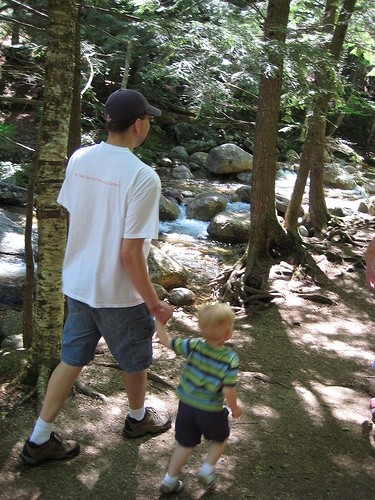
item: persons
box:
[366,235,375,418]
[155,304,243,494]
[19,89,174,466]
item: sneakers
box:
[196,472,217,491]
[19,431,80,468]
[122,407,171,438]
[370,397,375,416]
[160,480,184,494]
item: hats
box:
[104,89,162,123]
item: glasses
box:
[140,115,155,122]
